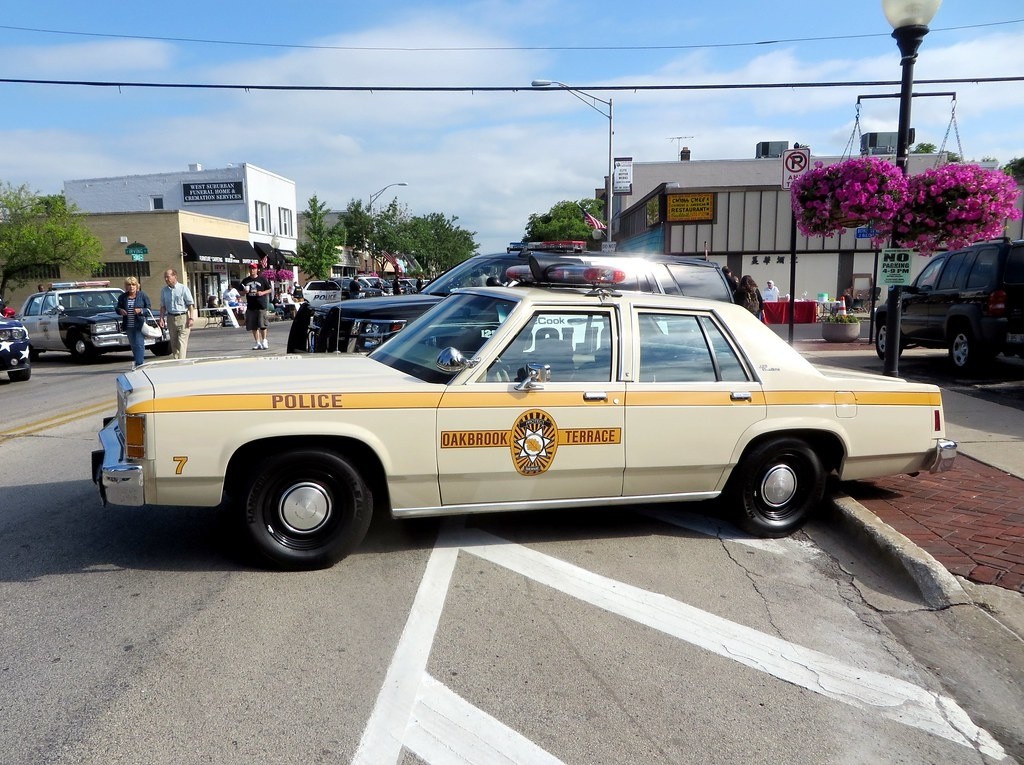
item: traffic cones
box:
[838,296,848,317]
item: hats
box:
[248,262,257,269]
[767,281,774,285]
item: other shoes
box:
[262,341,268,349]
[252,343,262,350]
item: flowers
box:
[260,269,277,281]
[276,268,295,282]
[790,155,912,250]
[894,161,1024,259]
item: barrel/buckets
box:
[818,293,828,301]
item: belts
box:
[168,312,187,316]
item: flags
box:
[578,206,607,231]
[382,251,397,266]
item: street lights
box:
[880,0,941,380]
[370,182,408,274]
[531,80,614,242]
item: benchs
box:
[640,342,731,381]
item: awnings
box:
[182,233,261,264]
[253,242,285,264]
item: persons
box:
[239,264,272,349]
[208,285,241,327]
[348,276,362,298]
[273,281,305,321]
[115,277,151,365]
[38,283,44,292]
[159,269,194,359]
[374,277,424,294]
[722,266,779,322]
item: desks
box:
[816,300,841,323]
[275,301,307,321]
[761,301,817,325]
[199,306,242,330]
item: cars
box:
[355,275,432,296]
[90,281,955,573]
[15,280,173,365]
[0,308,32,382]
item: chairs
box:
[569,346,611,381]
[530,338,574,381]
[206,303,223,329]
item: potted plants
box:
[822,313,864,342]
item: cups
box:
[245,286,250,293]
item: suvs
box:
[290,245,737,376]
[876,237,1024,380]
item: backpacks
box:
[294,285,302,295]
[742,290,759,313]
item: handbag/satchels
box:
[141,308,162,337]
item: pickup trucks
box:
[279,279,383,314]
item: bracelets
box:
[189,317,194,320]
